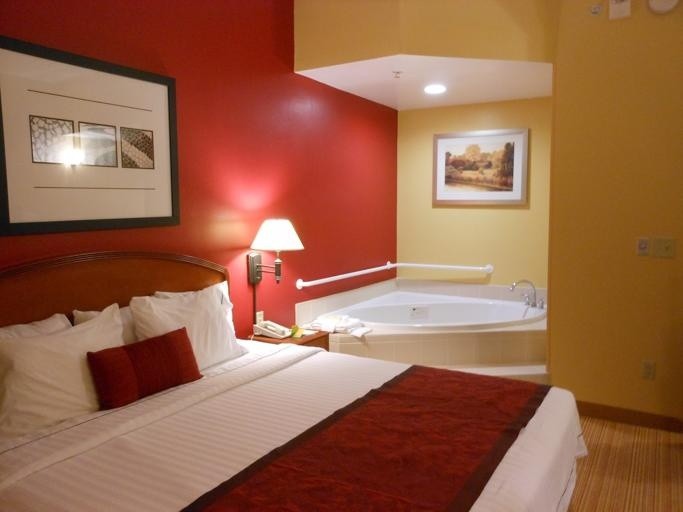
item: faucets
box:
[510,279,538,308]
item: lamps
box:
[249,219,305,281]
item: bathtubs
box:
[303,290,549,370]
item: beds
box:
[0,250,588,512]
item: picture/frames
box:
[0,36,180,237]
[432,127,529,205]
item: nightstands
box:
[247,329,329,352]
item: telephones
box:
[251,318,295,339]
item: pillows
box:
[154,280,235,340]
[130,287,249,371]
[0,313,72,342]
[72,306,138,345]
[0,303,125,438]
[87,327,204,410]
